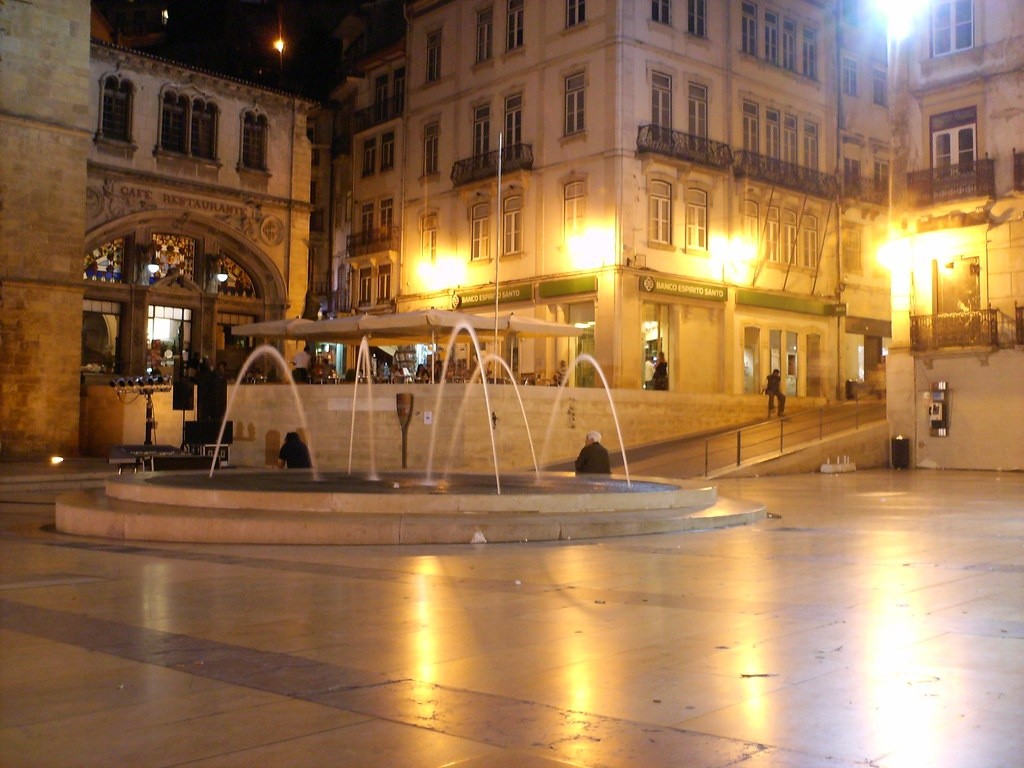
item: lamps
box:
[217,260,228,282]
[147,249,159,273]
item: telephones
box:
[929,403,943,421]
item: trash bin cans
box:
[846,378,858,399]
[891,434,910,471]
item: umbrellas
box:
[231,306,583,385]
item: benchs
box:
[108,444,177,475]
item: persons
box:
[645,351,667,390]
[288,345,314,382]
[390,365,401,383]
[416,364,429,384]
[760,369,786,417]
[575,431,610,473]
[277,432,313,469]
[434,360,447,382]
[556,360,569,387]
